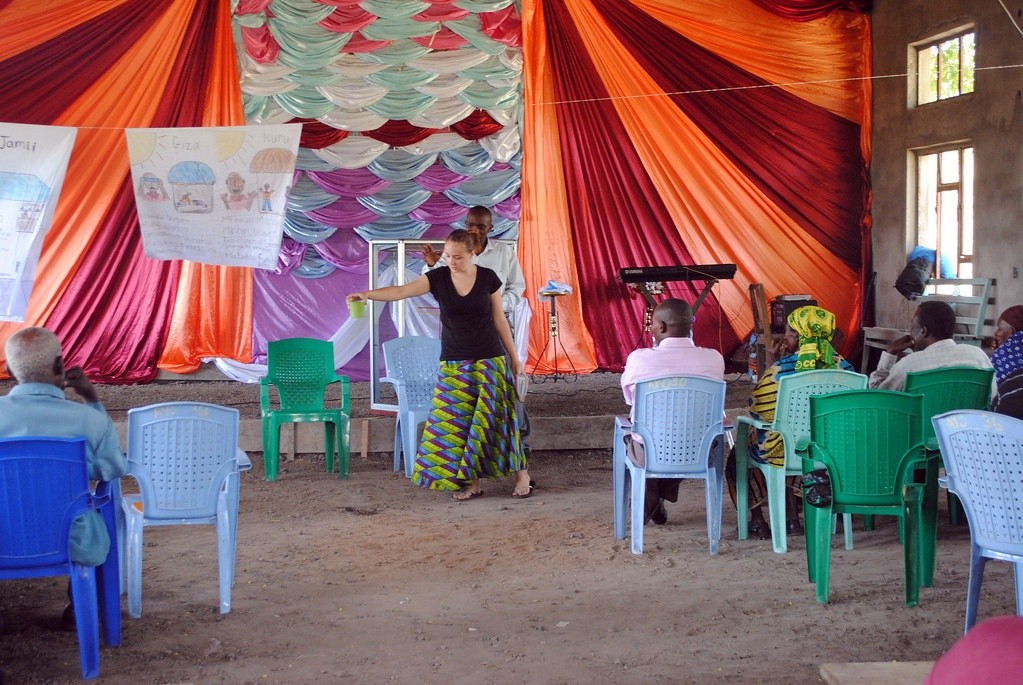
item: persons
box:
[0,327,130,632]
[992,306,1023,382]
[345,229,537,501]
[726,306,856,539]
[868,301,1000,483]
[620,299,725,525]
[421,205,532,456]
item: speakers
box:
[769,299,818,333]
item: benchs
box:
[860,278,993,377]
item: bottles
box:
[749,352,757,378]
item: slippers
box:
[514,480,536,499]
[451,487,484,502]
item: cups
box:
[349,302,364,318]
[753,374,758,383]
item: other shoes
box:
[61,606,78,632]
[644,496,660,525]
[786,519,803,537]
[650,500,667,523]
[736,520,769,538]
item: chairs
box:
[736,366,1023,638]
[117,400,253,615]
[258,338,352,480]
[612,372,737,555]
[382,335,443,477]
[0,438,121,678]
